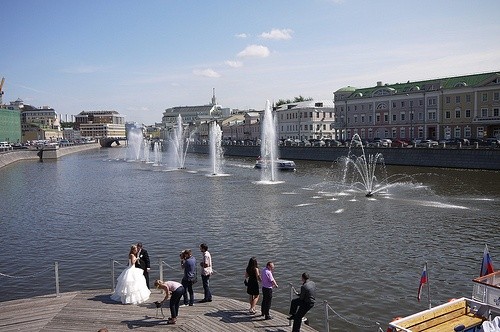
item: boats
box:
[255,155,296,172]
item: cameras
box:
[180,251,185,259]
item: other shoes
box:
[250,309,256,314]
[169,317,177,320]
[265,316,272,320]
[168,320,176,324]
[200,298,212,303]
[190,303,193,306]
[287,316,296,320]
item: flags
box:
[417,260,428,303]
[479,244,494,278]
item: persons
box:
[179,249,197,306]
[154,280,184,324]
[286,271,315,332]
[261,262,278,320]
[244,256,263,313]
[200,243,213,302]
[134,243,151,289]
[110,243,151,305]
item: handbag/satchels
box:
[203,267,213,276]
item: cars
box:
[185,137,500,149]
[0,137,94,149]
